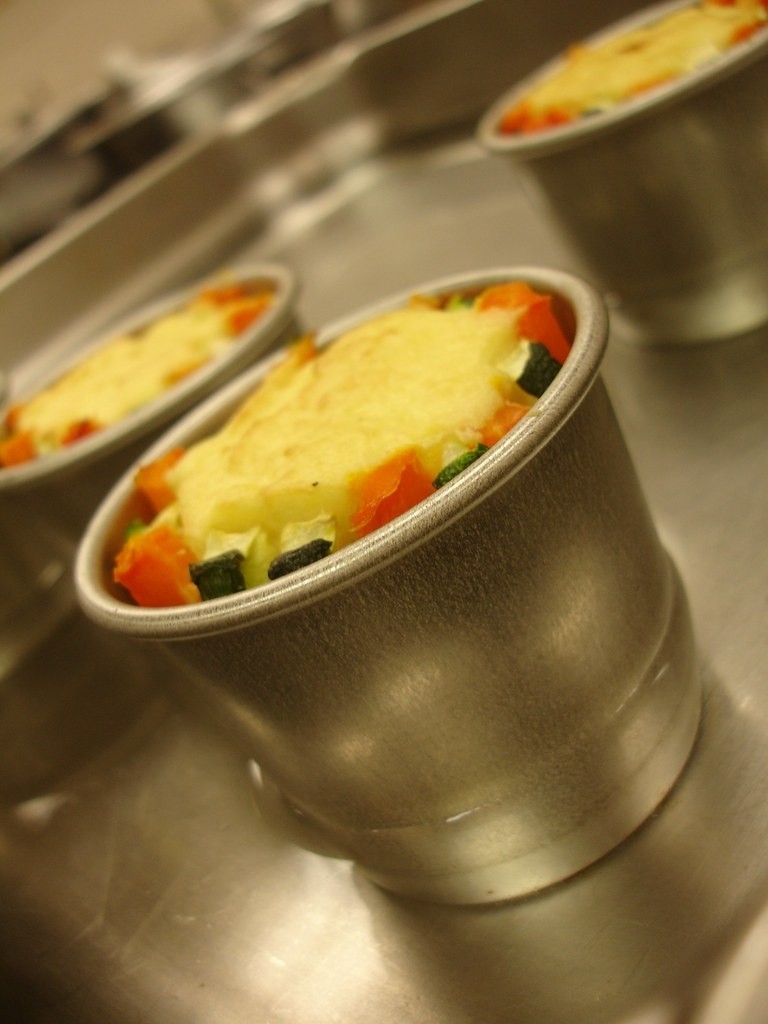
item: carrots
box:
[492,0,768,139]
[1,278,570,606]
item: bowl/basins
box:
[473,0,767,343]
[72,265,676,837]
[2,265,302,538]
